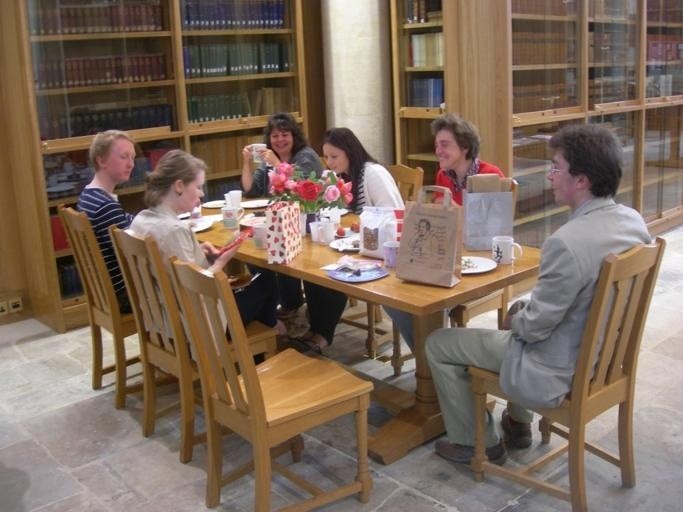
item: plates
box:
[461,253,498,276]
[330,238,361,252]
[330,265,387,283]
[177,200,224,233]
[333,227,360,237]
[241,199,347,227]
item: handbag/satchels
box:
[264,196,304,265]
[394,182,466,290]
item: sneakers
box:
[500,405,533,449]
[433,436,508,466]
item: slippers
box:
[286,330,332,359]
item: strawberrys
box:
[351,223,359,232]
[336,228,345,236]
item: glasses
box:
[548,167,571,175]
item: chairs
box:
[338,165,425,359]
[107,223,280,464]
[56,203,179,409]
[391,177,519,376]
[166,254,375,512]
[469,235,667,512]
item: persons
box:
[81,128,136,314]
[129,149,311,367]
[242,115,324,320]
[287,127,406,357]
[408,219,439,263]
[382,113,506,355]
[425,121,652,465]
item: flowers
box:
[267,163,353,214]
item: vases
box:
[306,214,316,233]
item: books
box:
[26,0,291,138]
[46,149,168,297]
[409,1,681,216]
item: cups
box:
[253,223,270,249]
[252,142,268,161]
[317,223,335,244]
[491,235,523,265]
[219,206,244,229]
[383,240,400,267]
[223,190,242,207]
[309,222,318,241]
